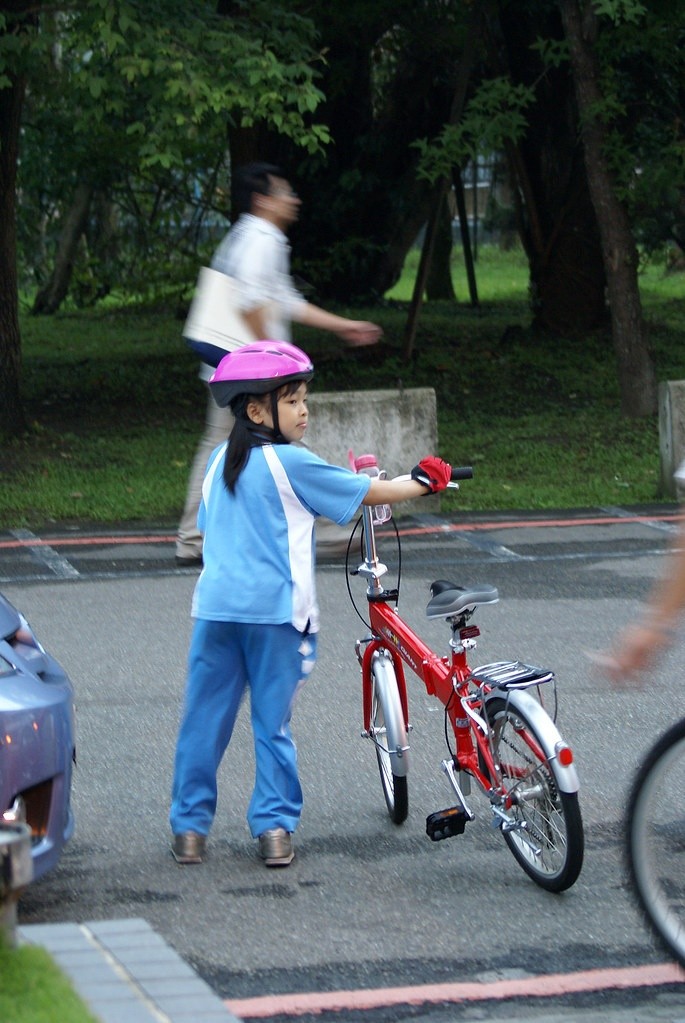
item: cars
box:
[0,590,77,894]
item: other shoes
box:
[174,554,207,568]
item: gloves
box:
[411,455,452,496]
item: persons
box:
[175,161,384,564]
[168,341,454,865]
[608,457,685,674]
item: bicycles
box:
[623,712,685,970]
[344,449,587,895]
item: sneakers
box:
[169,830,207,863]
[254,827,295,865]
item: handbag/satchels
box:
[182,266,260,368]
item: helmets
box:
[208,339,316,408]
